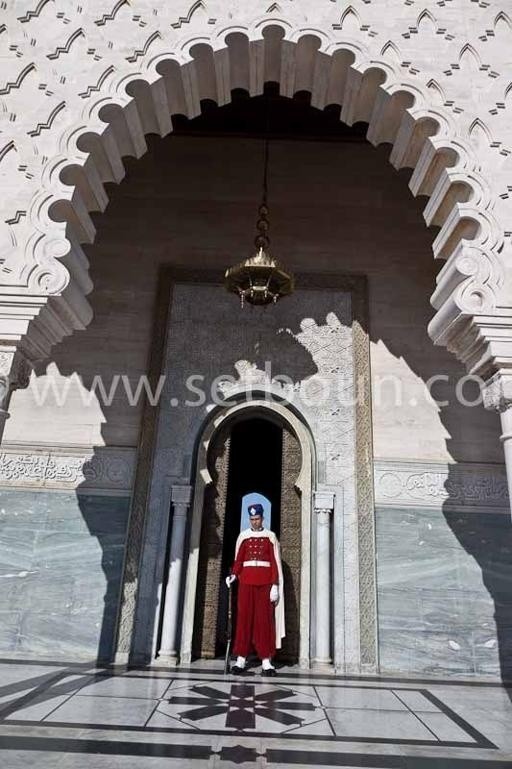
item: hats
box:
[248,504,263,516]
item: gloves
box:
[225,574,236,588]
[269,583,279,603]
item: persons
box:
[225,503,286,677]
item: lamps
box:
[224,139,293,308]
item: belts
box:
[242,560,271,568]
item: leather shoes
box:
[231,665,248,675]
[262,667,276,677]
